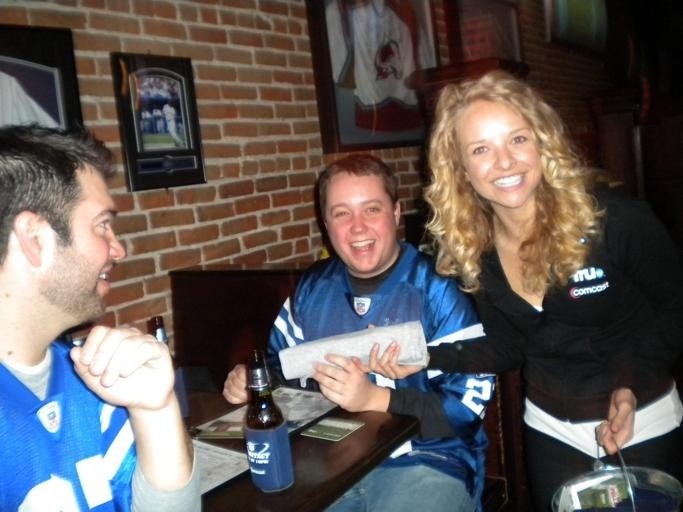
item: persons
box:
[224,153,497,512]
[141,102,177,132]
[374,71,683,512]
[1,122,202,512]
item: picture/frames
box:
[110,53,206,193]
[1,23,84,136]
[305,0,443,155]
[543,0,610,62]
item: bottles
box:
[152,316,190,421]
[241,349,295,495]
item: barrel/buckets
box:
[551,424,682,511]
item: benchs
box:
[168,267,526,512]
